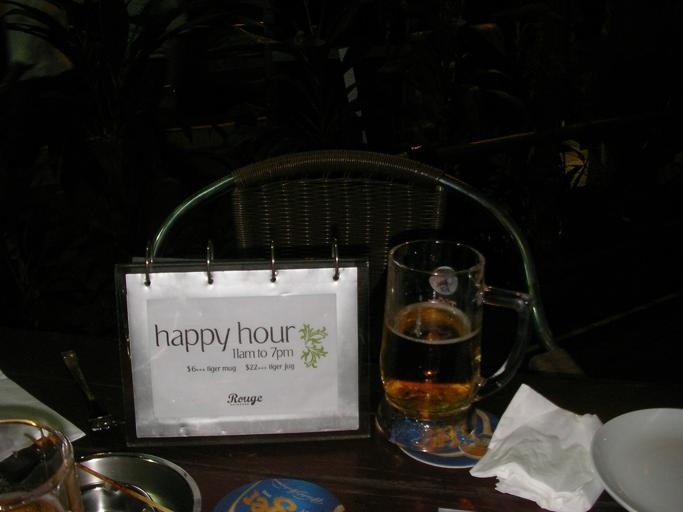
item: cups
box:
[380,238,533,424]
[0,417,84,512]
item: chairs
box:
[151,150,556,376]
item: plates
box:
[591,405,683,512]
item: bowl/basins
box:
[75,450,202,512]
